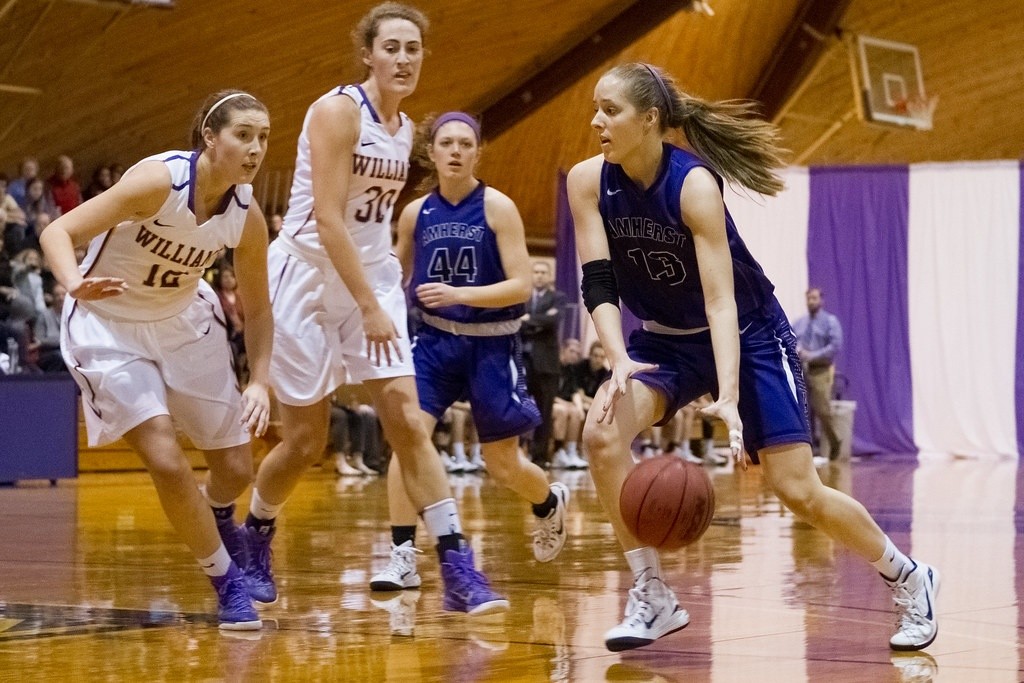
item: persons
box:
[0,154,841,477]
[236,6,513,620]
[367,114,570,594]
[43,89,283,632]
[561,63,941,652]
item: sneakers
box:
[533,481,570,562]
[219,519,250,572]
[440,539,510,615]
[240,523,278,606]
[205,561,261,630]
[604,566,690,652]
[370,540,425,589]
[334,439,726,475]
[879,555,940,650]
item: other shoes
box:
[828,437,844,461]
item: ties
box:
[536,290,540,311]
[802,319,813,349]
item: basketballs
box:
[618,454,718,550]
